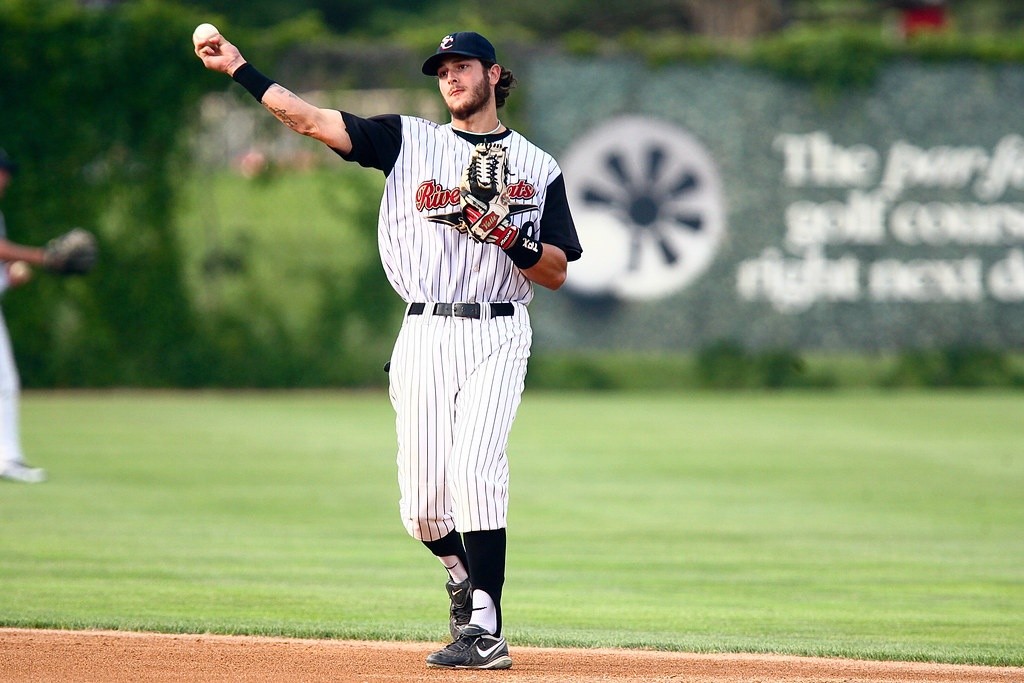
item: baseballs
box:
[193,22,218,47]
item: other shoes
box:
[1,461,46,486]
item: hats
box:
[0,146,26,172]
[421,31,500,76]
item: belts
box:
[407,302,515,320]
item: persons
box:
[194,24,585,673]
[0,146,100,483]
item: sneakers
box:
[422,624,512,671]
[444,575,475,640]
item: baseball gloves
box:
[43,227,97,275]
[461,141,517,245]
